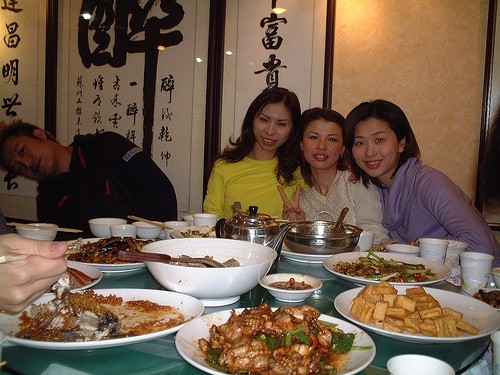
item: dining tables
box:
[0,256,493,375]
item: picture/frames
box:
[0,0,336,225]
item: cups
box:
[183,213,218,226]
[357,230,375,251]
[418,237,499,297]
[386,330,500,375]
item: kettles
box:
[215,202,296,271]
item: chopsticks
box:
[7,221,83,232]
[128,215,173,230]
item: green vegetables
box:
[336,251,437,282]
[203,319,373,375]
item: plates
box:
[279,241,360,263]
[45,262,104,292]
[2,288,205,351]
[171,226,215,238]
[334,286,499,345]
[323,252,452,288]
[175,308,376,375]
[65,238,157,273]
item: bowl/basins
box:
[384,243,420,256]
[89,218,188,238]
[280,219,364,254]
[259,273,323,302]
[141,237,278,307]
[16,222,58,240]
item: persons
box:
[342,99,500,268]
[0,118,178,231]
[203,86,310,220]
[0,233,70,313]
[277,107,390,246]
